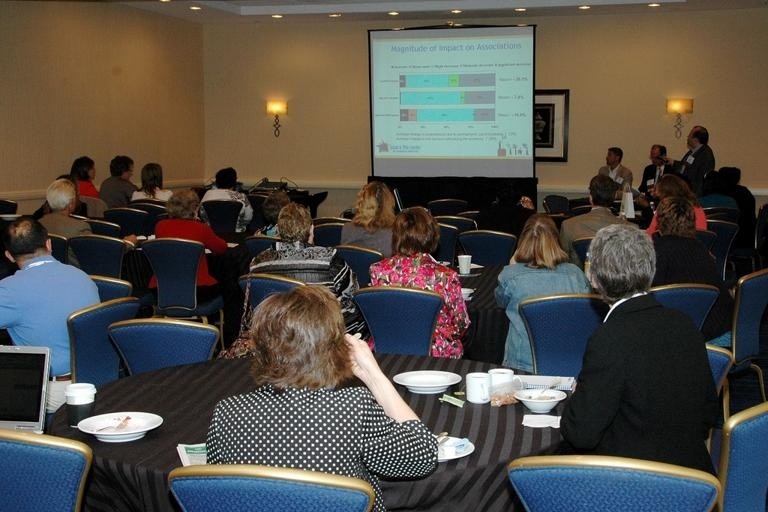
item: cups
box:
[457,254,474,276]
[466,369,524,403]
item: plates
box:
[392,370,462,394]
[431,432,478,464]
[77,411,165,443]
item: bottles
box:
[581,250,593,283]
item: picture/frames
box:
[533,89,570,164]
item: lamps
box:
[264,98,289,139]
[665,95,693,141]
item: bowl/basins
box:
[65,383,96,405]
[515,389,567,414]
[459,286,475,301]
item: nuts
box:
[491,394,518,407]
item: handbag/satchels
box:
[619,183,635,219]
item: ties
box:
[654,168,663,191]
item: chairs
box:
[0,169,766,511]
